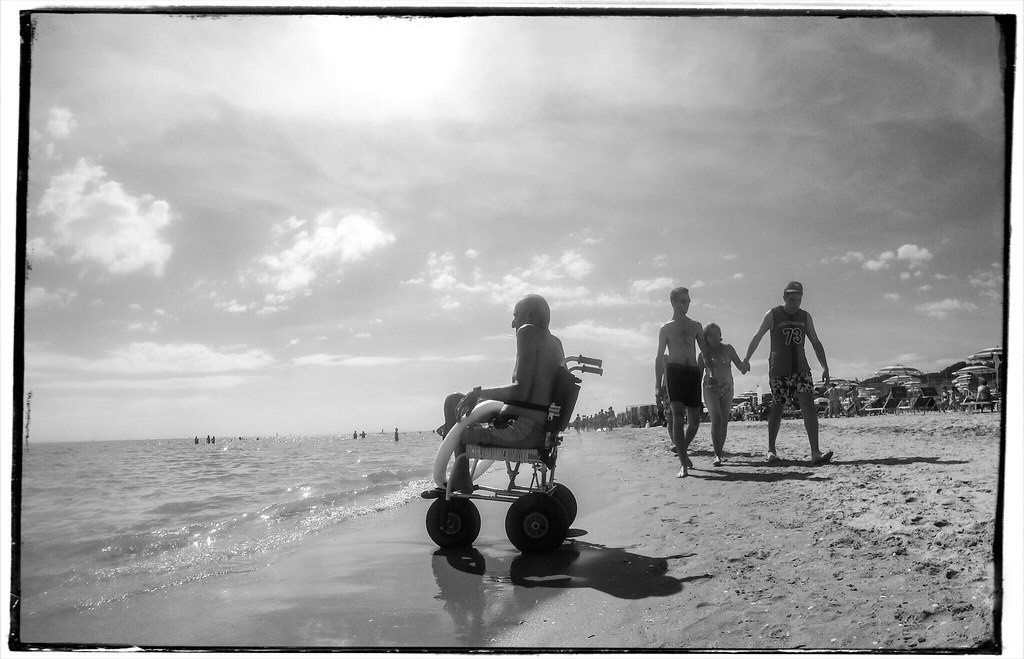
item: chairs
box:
[865,386,908,416]
[960,388,993,412]
[899,386,939,416]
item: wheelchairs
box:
[426,354,603,556]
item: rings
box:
[457,408,462,412]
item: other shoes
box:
[670,443,678,457]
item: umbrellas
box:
[873,363,926,397]
[951,345,1002,399]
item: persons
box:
[420,295,569,500]
[655,286,718,478]
[655,354,692,455]
[568,404,667,434]
[812,381,909,418]
[352,427,436,442]
[700,383,803,422]
[697,321,751,465]
[193,433,260,445]
[916,380,1000,412]
[740,281,834,467]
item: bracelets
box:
[473,385,483,401]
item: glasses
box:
[680,299,690,303]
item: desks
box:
[991,393,1002,411]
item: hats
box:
[784,281,803,293]
